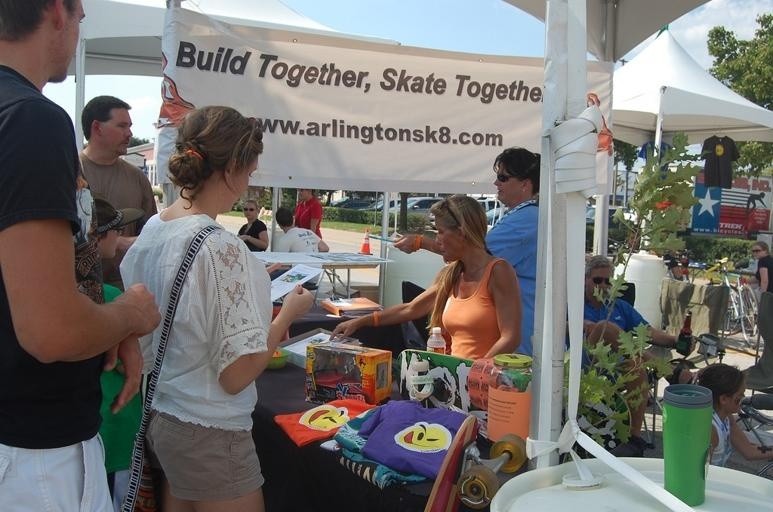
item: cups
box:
[661,386,713,506]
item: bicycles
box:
[706,258,761,350]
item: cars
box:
[584,203,627,224]
[330,197,510,222]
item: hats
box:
[89,197,147,236]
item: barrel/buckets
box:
[614,253,664,329]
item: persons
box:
[119,106,315,511]
[295,188,322,238]
[77,95,157,290]
[329,198,523,359]
[698,364,772,468]
[752,241,773,295]
[394,148,540,354]
[1,1,162,511]
[266,209,329,288]
[566,256,695,449]
[237,201,268,252]
[94,197,142,509]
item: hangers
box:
[703,126,734,146]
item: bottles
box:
[427,327,446,354]
[360,228,371,255]
[676,310,693,355]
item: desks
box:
[255,253,392,308]
[288,298,384,342]
[246,360,526,512]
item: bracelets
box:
[415,237,422,250]
[372,311,380,327]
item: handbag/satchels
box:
[121,436,159,512]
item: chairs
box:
[401,281,431,344]
[739,288,772,451]
[616,283,659,448]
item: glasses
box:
[751,248,764,253]
[242,207,254,212]
[496,173,515,183]
[590,275,613,285]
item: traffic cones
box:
[358,228,374,255]
